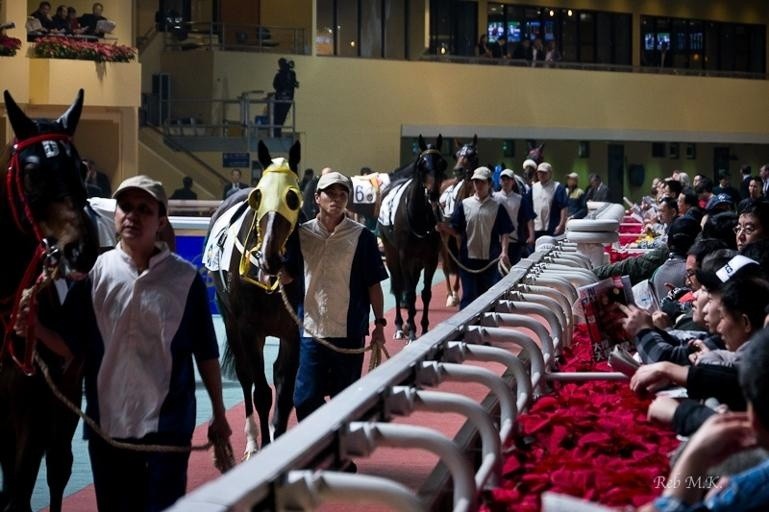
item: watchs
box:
[373,317,387,328]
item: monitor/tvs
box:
[524,19,556,43]
[675,32,704,52]
[644,32,672,51]
[488,18,521,44]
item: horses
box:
[302,159,418,223]
[0,87,177,512]
[203,138,305,461]
[440,133,480,308]
[376,134,448,344]
[521,142,545,187]
[487,161,530,195]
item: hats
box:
[500,158,581,181]
[316,171,351,193]
[471,167,491,181]
[112,174,167,204]
[666,193,760,284]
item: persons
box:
[270,55,300,133]
[585,173,613,201]
[523,159,541,188]
[84,161,110,197]
[297,166,387,261]
[491,168,538,267]
[434,166,516,311]
[165,176,197,200]
[278,171,389,424]
[585,159,768,512]
[566,172,590,220]
[11,170,235,510]
[21,2,113,42]
[223,169,248,201]
[525,161,571,255]
[401,23,723,71]
